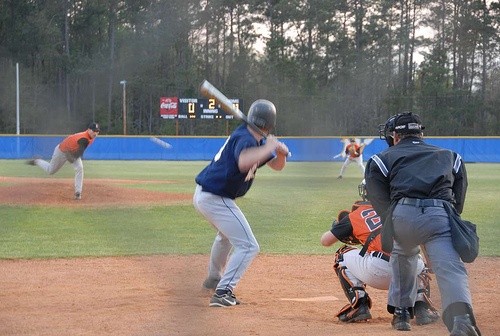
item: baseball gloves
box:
[349,146,355,153]
[330,208,363,245]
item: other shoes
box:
[338,176,342,179]
[390,307,411,332]
[25,158,37,165]
[449,314,481,336]
[73,192,82,200]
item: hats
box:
[388,111,425,137]
[349,138,355,142]
[88,122,100,131]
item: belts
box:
[398,197,451,208]
[373,251,390,262]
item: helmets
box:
[247,98,276,129]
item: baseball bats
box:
[198,79,292,158]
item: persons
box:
[320,180,440,324]
[364,112,482,336]
[337,137,365,179]
[193,99,289,306]
[26,122,100,200]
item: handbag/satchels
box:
[380,201,397,252]
[442,202,480,263]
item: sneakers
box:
[414,301,441,326]
[202,278,222,290]
[209,290,240,307]
[338,297,372,323]
